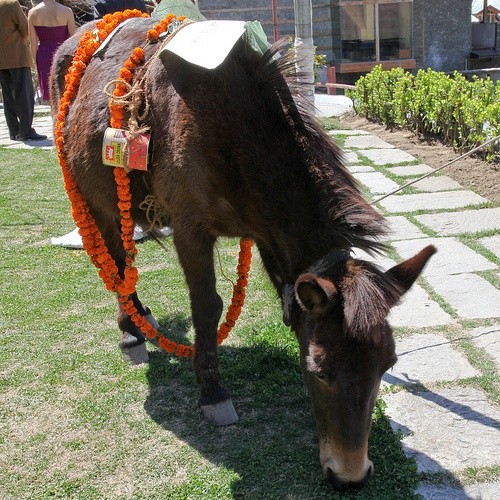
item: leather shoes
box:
[16,131,48,141]
[9,132,19,139]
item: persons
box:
[0,1,48,141]
[27,0,77,101]
[150,0,207,22]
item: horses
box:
[47,15,439,493]
